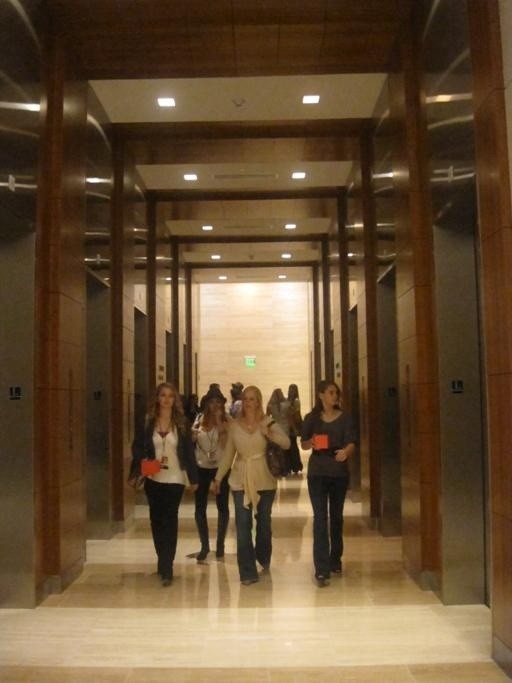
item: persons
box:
[300,380,357,588]
[210,385,292,585]
[184,384,304,491]
[190,388,234,560]
[131,382,199,588]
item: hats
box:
[232,383,243,390]
[201,388,226,409]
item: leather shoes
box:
[242,562,270,584]
[158,568,171,586]
[316,567,341,579]
[196,550,209,560]
[216,543,224,556]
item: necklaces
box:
[157,416,172,449]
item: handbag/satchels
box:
[266,420,292,477]
